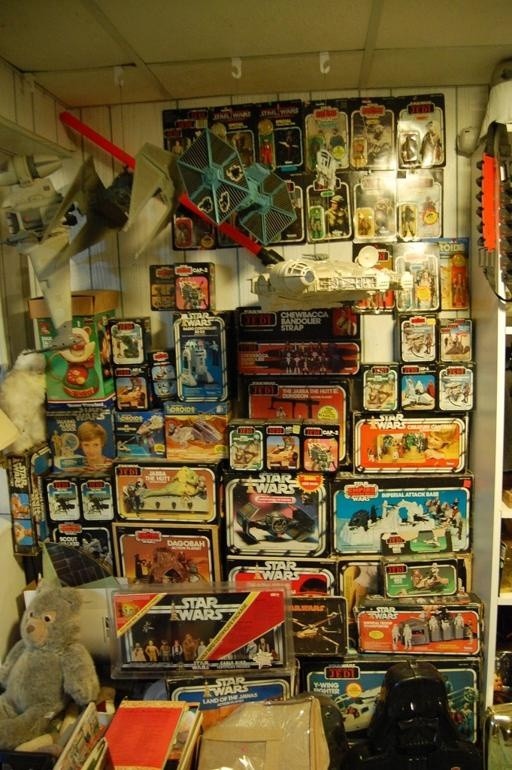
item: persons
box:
[424,423,459,464]
[132,635,271,664]
[82,425,116,472]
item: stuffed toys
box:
[1,581,101,752]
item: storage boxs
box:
[53,700,201,769]
